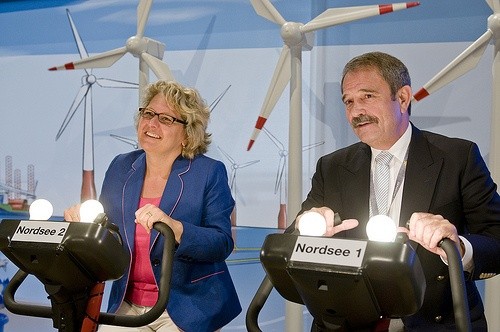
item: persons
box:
[64,82,242,332]
[284,51,500,332]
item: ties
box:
[370,153,405,214]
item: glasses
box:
[138,107,185,126]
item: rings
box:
[146,211,151,216]
[70,213,78,217]
